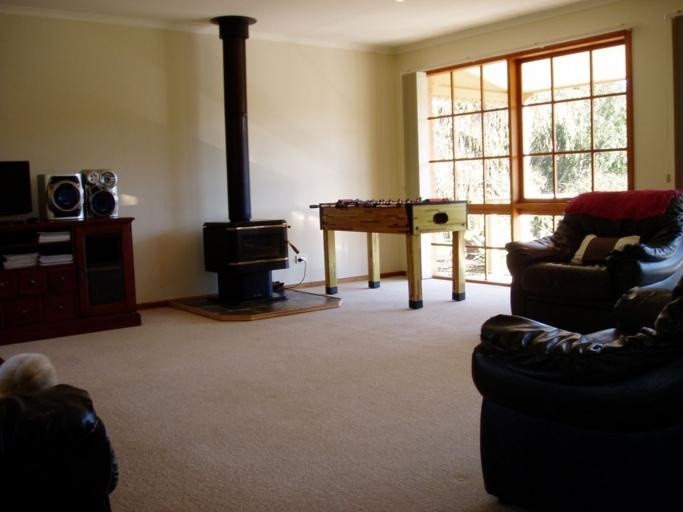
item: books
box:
[3,253,75,270]
[37,232,70,243]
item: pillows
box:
[571,234,641,267]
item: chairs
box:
[471,268,683,509]
[503,188,683,339]
[0,353,121,512]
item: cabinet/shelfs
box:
[1,218,143,345]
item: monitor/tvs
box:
[0,160,34,217]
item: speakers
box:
[37,172,84,221]
[79,168,120,219]
